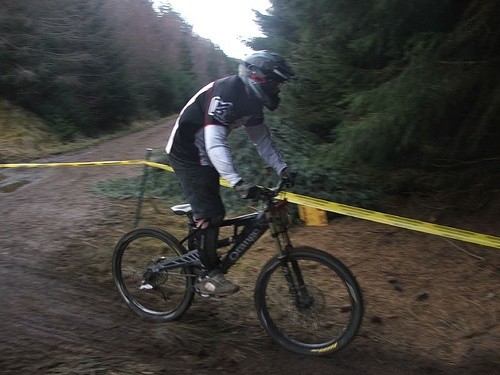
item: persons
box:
[166,49,301,297]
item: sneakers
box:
[199,273,240,296]
[180,265,206,277]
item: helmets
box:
[237,50,294,112]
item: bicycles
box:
[112,169,364,357]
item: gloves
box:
[234,180,261,201]
[281,167,295,188]
